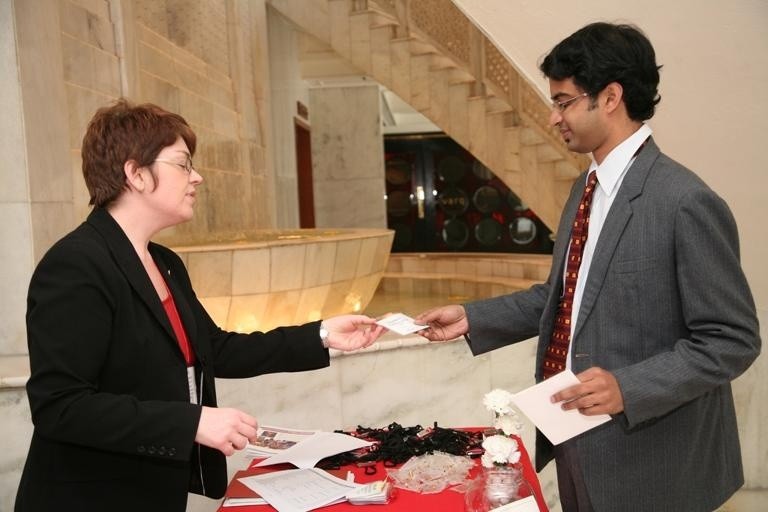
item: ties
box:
[541,171,598,382]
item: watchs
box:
[319,320,330,348]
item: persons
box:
[14,96,393,512]
[413,22,761,512]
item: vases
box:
[482,471,520,507]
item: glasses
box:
[550,92,590,112]
[154,156,195,173]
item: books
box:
[246,426,322,457]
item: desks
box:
[215,426,547,511]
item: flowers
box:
[480,434,522,470]
[482,387,525,436]
